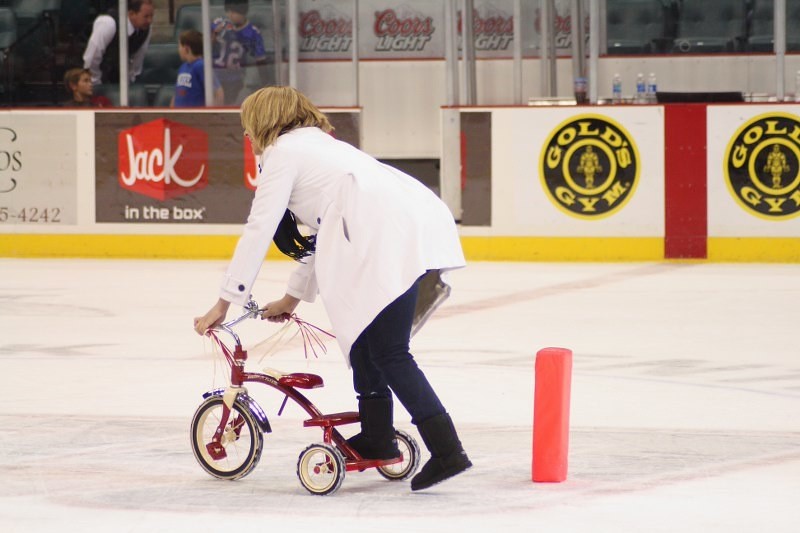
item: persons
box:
[63,0,155,107]
[210,0,274,105]
[193,86,476,491]
[169,29,225,108]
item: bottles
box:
[648,73,658,104]
[636,74,646,104]
[613,73,622,104]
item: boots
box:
[334,396,400,460]
[411,413,473,491]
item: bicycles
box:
[187,293,421,497]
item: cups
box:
[574,77,586,104]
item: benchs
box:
[0,1,287,111]
[605,0,800,54]
